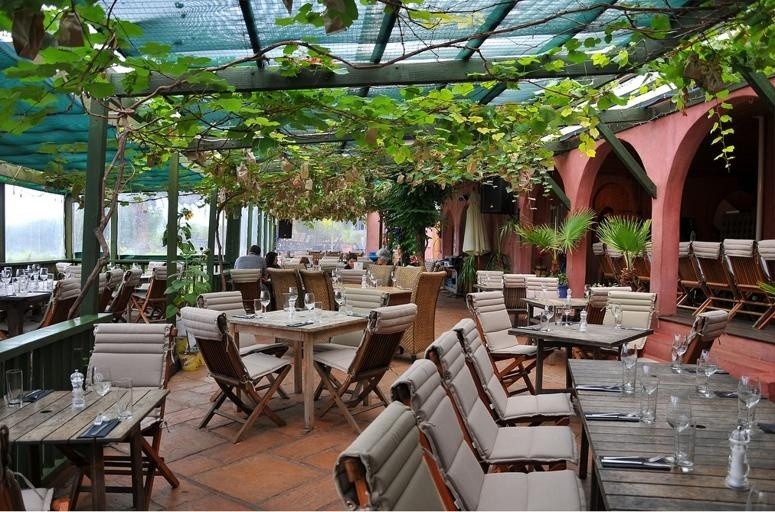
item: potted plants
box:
[512,207,597,287]
[591,213,657,293]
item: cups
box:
[40,268,48,290]
[738,399,756,436]
[32,263,40,278]
[12,277,20,294]
[620,341,638,395]
[391,271,397,288]
[47,273,53,290]
[283,293,290,311]
[672,343,682,370]
[114,375,134,421]
[563,301,571,328]
[332,269,338,289]
[544,302,554,333]
[696,358,707,393]
[666,394,688,466]
[637,360,660,424]
[640,383,657,421]
[27,265,34,286]
[541,312,549,332]
[93,367,111,424]
[304,292,316,323]
[668,329,690,373]
[699,349,719,399]
[623,359,636,394]
[260,290,270,318]
[556,307,563,328]
[254,299,262,315]
[335,290,344,316]
[611,300,620,326]
[314,301,322,321]
[6,369,24,408]
[288,287,298,302]
[678,417,696,473]
[16,268,25,289]
[2,269,11,294]
[737,376,762,439]
[5,266,12,280]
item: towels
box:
[575,382,621,391]
[584,409,641,422]
[232,312,256,319]
[600,453,674,471]
[286,319,314,327]
[757,421,775,434]
[517,324,543,331]
[7,387,55,403]
[684,365,729,376]
[713,389,739,398]
[623,325,651,333]
[347,310,368,318]
[76,416,120,439]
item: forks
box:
[575,385,626,392]
[603,451,669,463]
[578,382,619,389]
[592,410,640,418]
[29,390,44,399]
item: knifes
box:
[91,420,113,436]
[25,389,41,397]
[599,458,679,468]
[584,414,642,420]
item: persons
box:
[344,254,360,270]
[264,252,280,267]
[235,245,267,279]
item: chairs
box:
[197,290,291,403]
[635,309,730,363]
[332,400,459,510]
[572,287,633,358]
[581,290,658,360]
[71,323,181,511]
[313,287,391,406]
[265,251,374,271]
[627,241,651,293]
[466,291,554,396]
[502,274,536,328]
[338,270,365,284]
[390,359,590,510]
[99,273,107,313]
[676,242,722,316]
[299,268,339,311]
[125,266,182,322]
[593,243,620,286]
[524,276,563,350]
[362,265,395,288]
[230,269,270,314]
[607,242,647,292]
[0,330,11,342]
[131,261,167,309]
[723,239,775,329]
[423,330,578,472]
[692,241,756,320]
[181,306,293,444]
[62,265,81,279]
[104,268,143,323]
[452,317,576,427]
[646,242,651,264]
[100,268,123,313]
[0,424,73,511]
[54,263,74,280]
[393,266,422,290]
[267,267,305,311]
[313,303,418,436]
[177,262,186,278]
[391,271,447,364]
[476,270,504,292]
[756,240,775,330]
[37,278,81,330]
